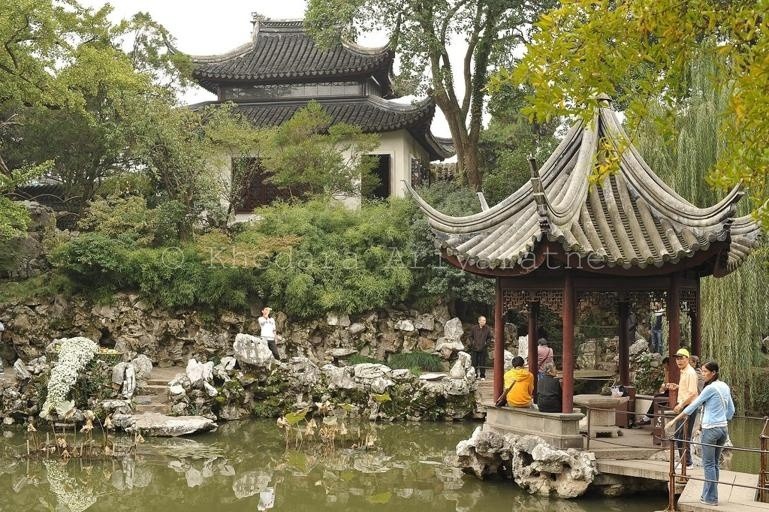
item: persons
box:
[664,361,735,506]
[537,338,553,378]
[651,301,666,356]
[666,348,697,470]
[537,363,561,412]
[468,316,491,382]
[258,305,281,362]
[504,355,534,409]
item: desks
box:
[574,394,629,437]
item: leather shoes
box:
[636,419,651,425]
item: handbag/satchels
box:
[495,394,507,406]
[691,430,733,468]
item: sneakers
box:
[674,462,693,469]
[700,495,718,506]
[481,378,485,381]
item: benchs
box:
[481,402,584,441]
[635,395,655,424]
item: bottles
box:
[657,383,673,393]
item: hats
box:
[674,349,690,358]
